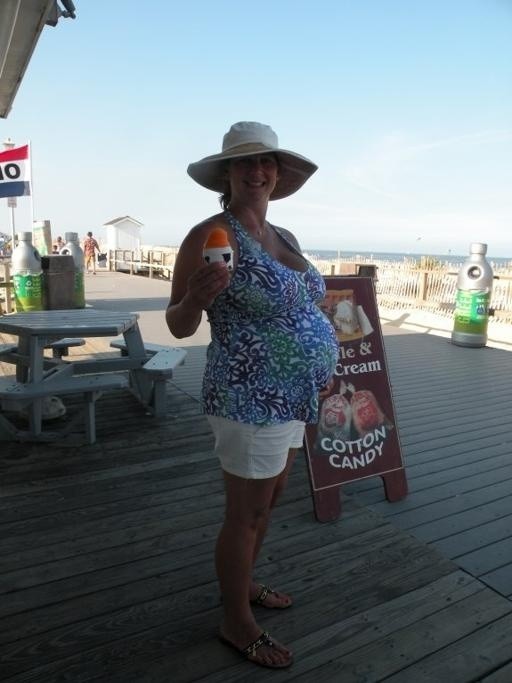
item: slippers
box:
[249,583,292,610]
[216,629,294,668]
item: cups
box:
[202,248,233,273]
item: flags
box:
[0,144,31,198]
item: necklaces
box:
[231,208,268,236]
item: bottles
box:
[59,232,87,309]
[10,233,47,312]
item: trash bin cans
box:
[95,252,107,268]
[12,232,85,313]
[339,263,379,288]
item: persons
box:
[164,121,338,669]
[0,229,100,274]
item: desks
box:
[0,309,152,439]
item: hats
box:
[187,121,318,200]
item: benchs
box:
[0,336,188,444]
